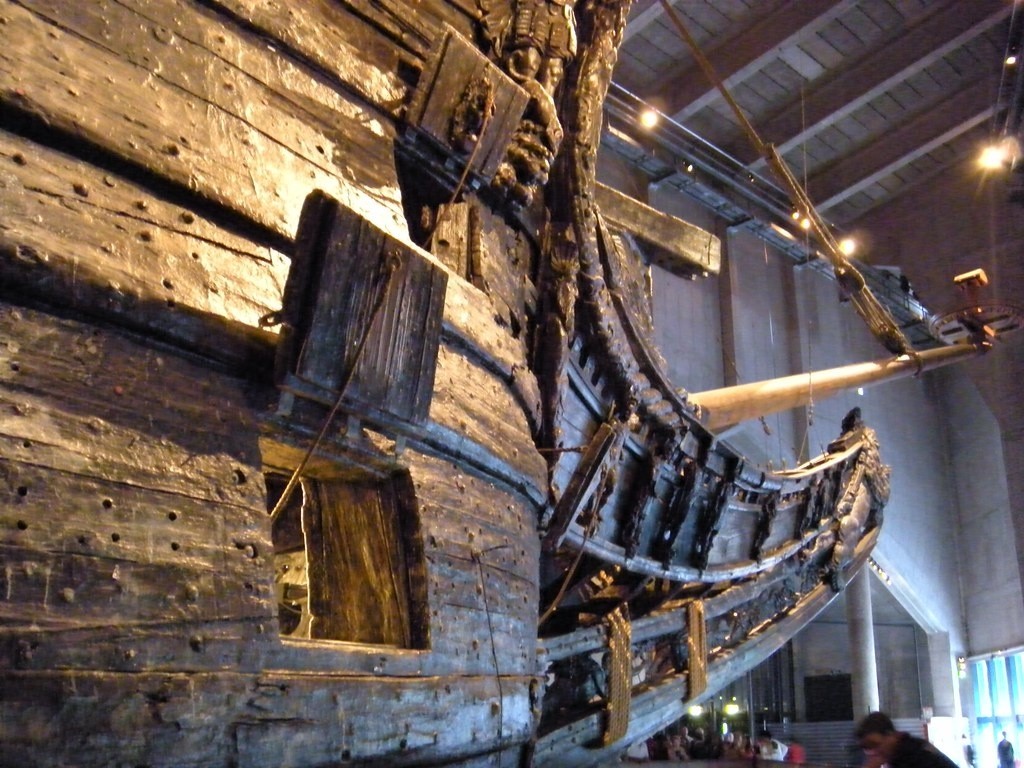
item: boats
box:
[0,1,1008,768]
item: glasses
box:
[858,737,884,750]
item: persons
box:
[626,725,806,768]
[853,711,1015,768]
